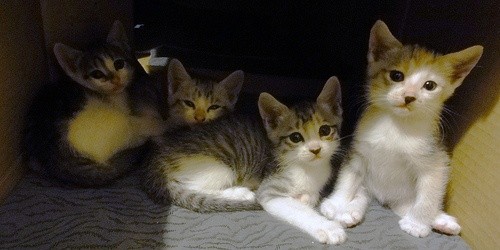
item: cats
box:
[138,75,348,246]
[318,19,485,238]
[27,18,167,189]
[168,58,311,129]
